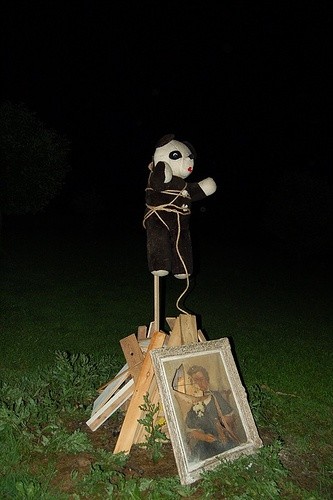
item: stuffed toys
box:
[146,133,217,279]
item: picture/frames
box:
[147,336,263,487]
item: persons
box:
[183,364,235,462]
